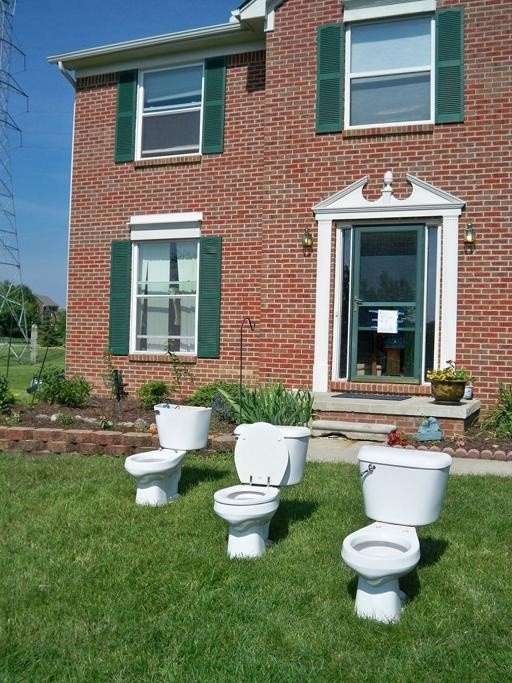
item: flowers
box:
[425,361,468,380]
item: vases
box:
[430,380,466,403]
[465,386,473,399]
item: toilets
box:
[341,443,452,624]
[213,420,311,559]
[124,401,212,509]
